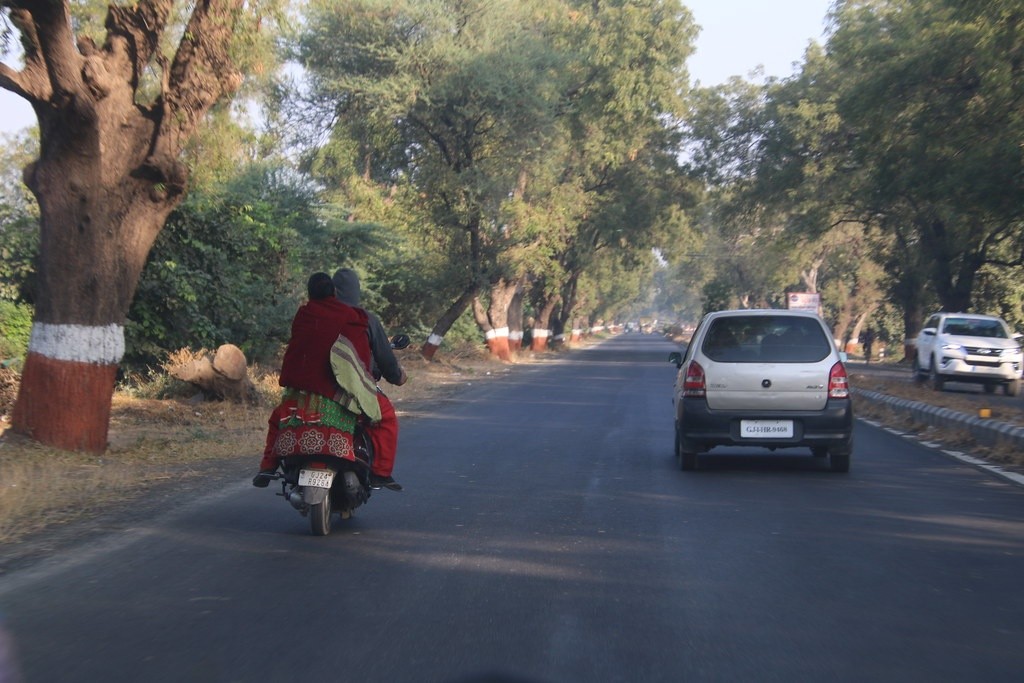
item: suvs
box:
[912,311,1024,398]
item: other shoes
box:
[371,474,402,492]
[253,470,274,488]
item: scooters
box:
[259,333,411,536]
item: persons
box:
[254,268,407,493]
[860,327,873,354]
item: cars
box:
[668,308,854,473]
